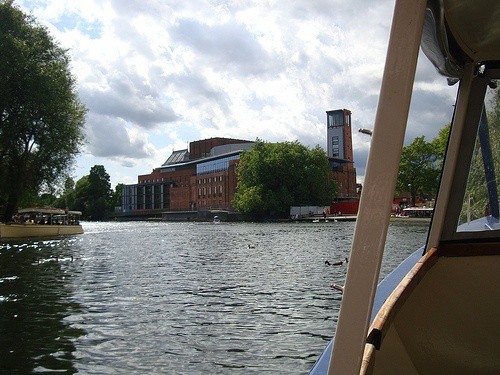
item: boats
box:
[0,208,83,239]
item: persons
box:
[321,208,342,217]
[11,214,79,226]
